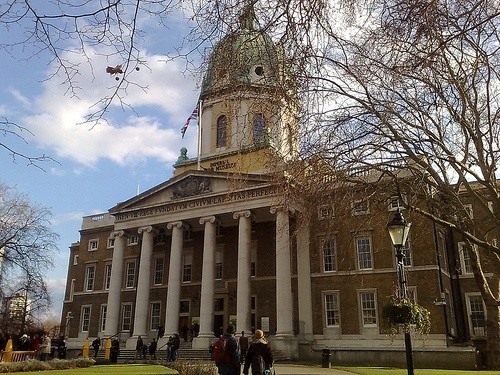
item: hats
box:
[226,326,235,333]
[242,331,244,333]
[254,330,264,338]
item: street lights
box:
[386,207,414,375]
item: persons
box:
[92,337,102,358]
[110,337,120,364]
[167,332,180,362]
[148,338,157,362]
[243,329,275,375]
[190,320,199,342]
[136,336,148,359]
[210,325,246,375]
[239,331,248,364]
[38,334,52,361]
[156,323,165,342]
[15,334,32,350]
[181,324,189,342]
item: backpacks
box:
[252,355,264,374]
[213,338,230,363]
